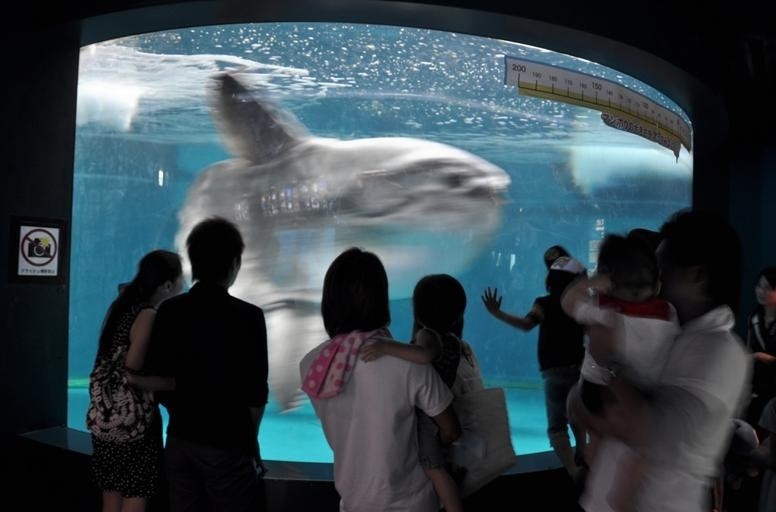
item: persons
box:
[558,206,748,512]
[477,244,593,481]
[138,214,271,511]
[83,248,183,511]
[356,270,475,510]
[294,244,462,512]
[721,266,775,512]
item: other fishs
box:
[75,44,330,134]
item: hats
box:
[544,244,590,302]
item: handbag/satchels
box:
[442,388,518,511]
[86,355,159,447]
